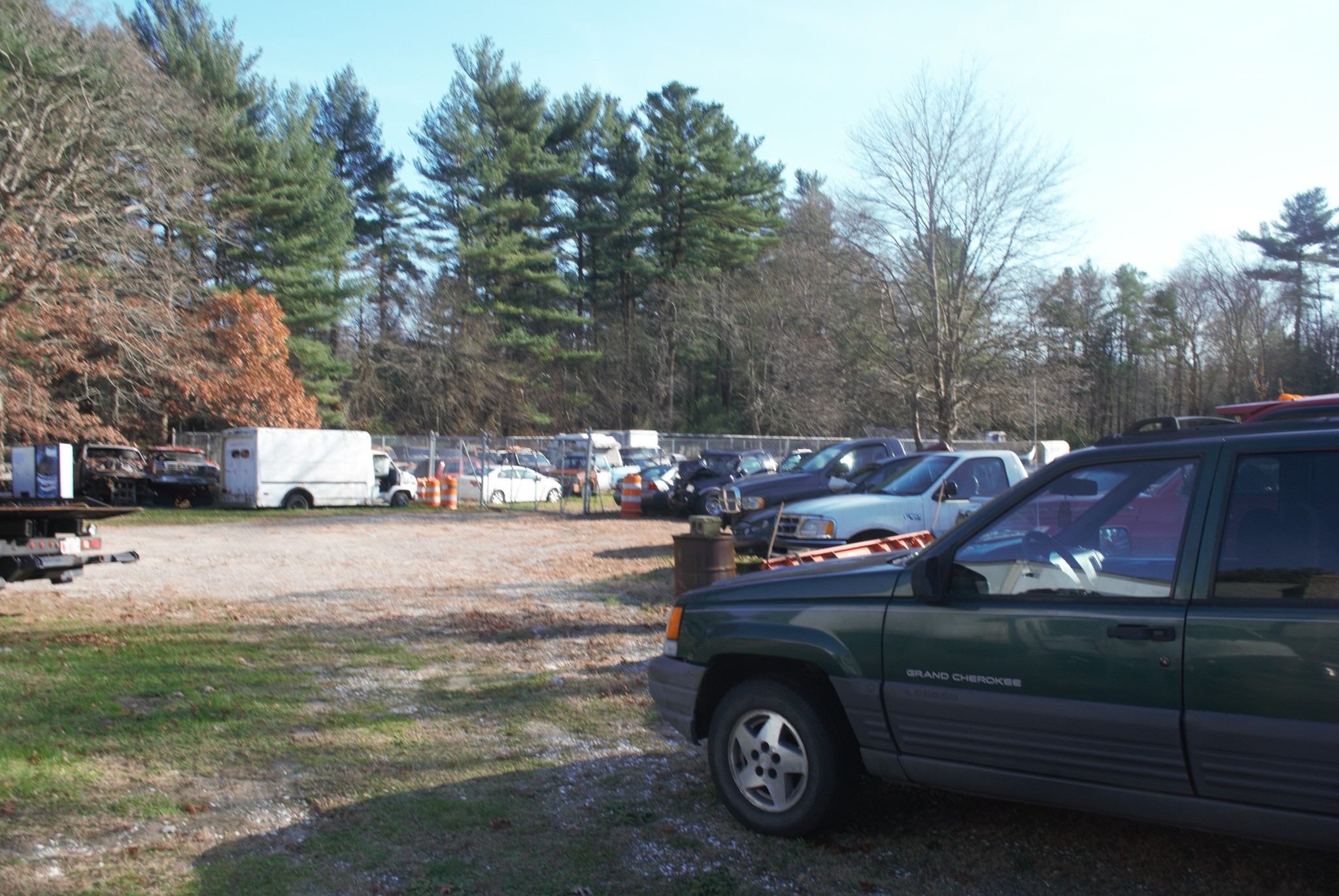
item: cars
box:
[373,446,610,503]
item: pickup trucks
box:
[614,391,1338,840]
[67,445,220,507]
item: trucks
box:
[217,427,415,512]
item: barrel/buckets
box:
[671,532,737,602]
